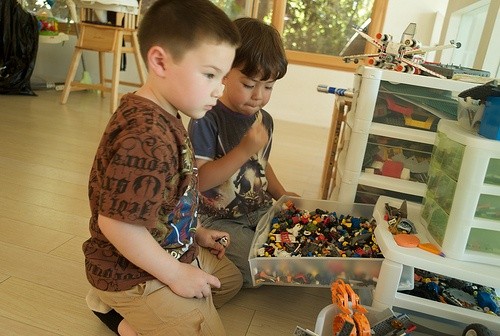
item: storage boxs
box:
[329,65,485,205]
[419,118,500,266]
[313,300,500,336]
[248,194,500,331]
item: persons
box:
[82,0,244,336]
[187,17,302,290]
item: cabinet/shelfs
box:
[59,20,148,114]
